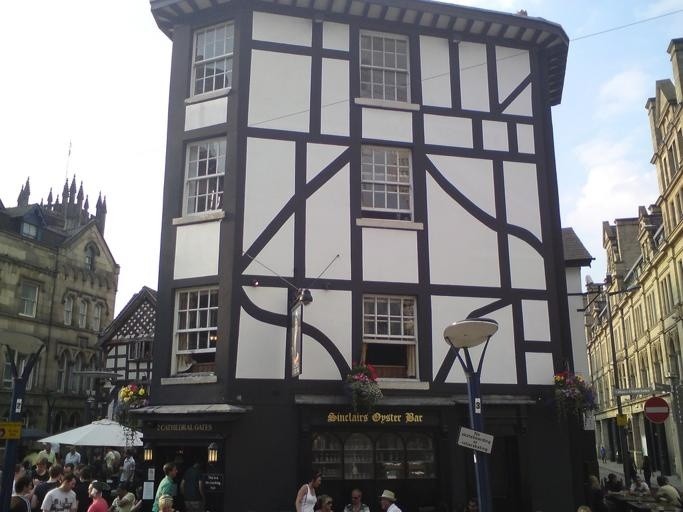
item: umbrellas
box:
[36,418,143,446]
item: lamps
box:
[207,440,222,473]
[175,448,184,457]
[142,442,158,471]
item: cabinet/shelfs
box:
[310,431,435,481]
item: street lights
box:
[74,369,123,482]
[605,282,644,491]
[0,329,48,512]
[441,316,500,512]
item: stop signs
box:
[643,397,670,424]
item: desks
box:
[611,493,683,512]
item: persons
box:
[150,462,178,512]
[180,455,206,512]
[378,489,401,512]
[294,472,323,512]
[314,494,334,512]
[343,488,371,512]
[10,443,145,512]
[158,494,179,512]
[600,446,683,507]
[465,495,478,512]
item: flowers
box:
[345,360,383,412]
[114,380,150,459]
[552,372,605,426]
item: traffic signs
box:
[613,387,653,394]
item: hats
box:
[376,489,398,501]
